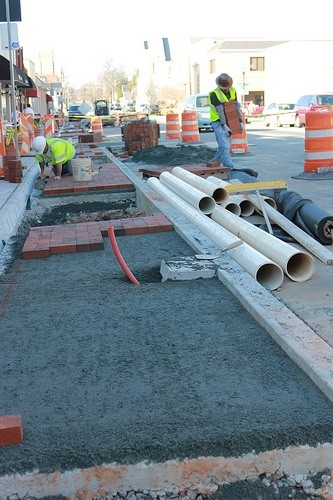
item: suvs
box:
[67,105,86,121]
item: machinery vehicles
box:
[242,101,264,116]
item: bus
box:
[184,95,217,131]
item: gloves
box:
[240,122,243,130]
[223,124,232,137]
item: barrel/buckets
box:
[71,158,92,181]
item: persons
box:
[209,73,244,171]
[11,103,36,129]
[33,136,78,180]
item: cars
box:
[263,94,333,128]
[110,103,160,115]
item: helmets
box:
[31,136,46,154]
[216,73,233,89]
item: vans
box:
[94,100,109,116]
